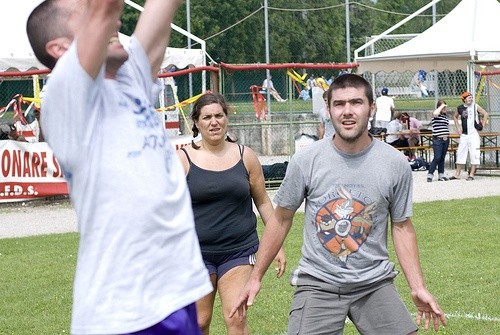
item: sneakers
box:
[427,173,432,182]
[438,173,446,181]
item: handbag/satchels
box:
[474,121,483,131]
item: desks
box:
[373,133,500,168]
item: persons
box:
[449,93,490,181]
[399,112,422,162]
[260,67,336,101]
[2,102,43,143]
[411,73,429,98]
[376,88,395,130]
[426,100,450,183]
[228,75,447,335]
[384,111,410,159]
[26,0,217,335]
[173,92,287,335]
[318,89,337,140]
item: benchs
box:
[380,87,422,98]
[396,146,500,171]
[260,90,282,95]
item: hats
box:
[461,92,471,100]
[381,88,388,95]
[0,125,10,134]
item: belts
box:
[433,135,450,140]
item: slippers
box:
[449,176,460,180]
[466,176,474,181]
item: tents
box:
[355,0,500,104]
[0,1,208,95]
